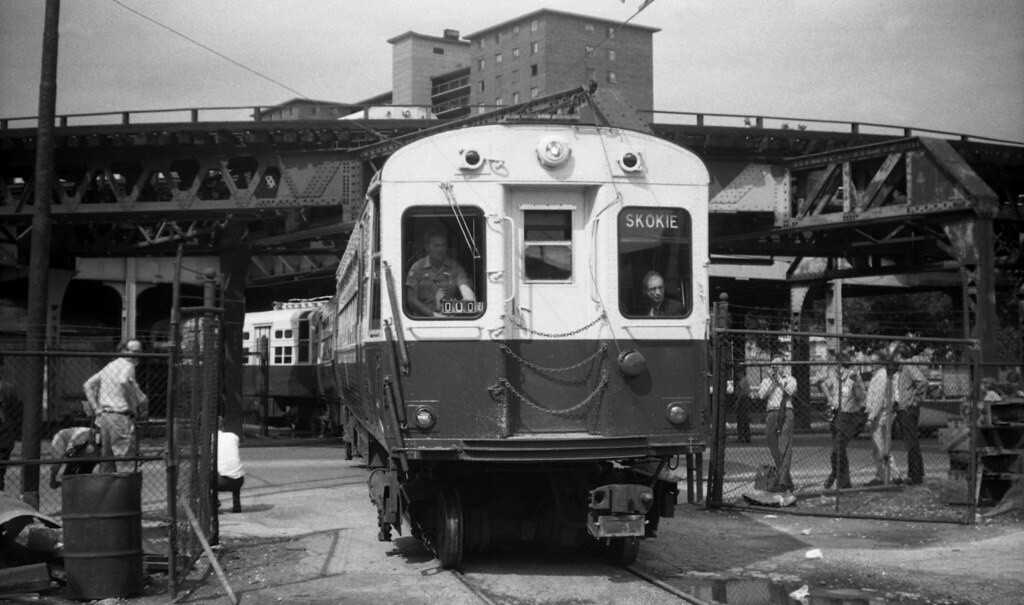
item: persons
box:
[758,353,797,493]
[820,353,865,489]
[83,338,149,473]
[731,361,754,443]
[0,350,25,492]
[642,270,687,317]
[211,415,245,513]
[48,418,103,490]
[405,229,477,319]
[980,376,1002,402]
[893,345,926,485]
[863,351,904,486]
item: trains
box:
[181,128,711,571]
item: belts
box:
[103,409,134,417]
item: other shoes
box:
[864,478,883,487]
[823,471,837,489]
[835,481,851,489]
[891,479,904,485]
[903,476,924,484]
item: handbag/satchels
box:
[754,464,779,492]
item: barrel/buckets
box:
[62,473,144,600]
